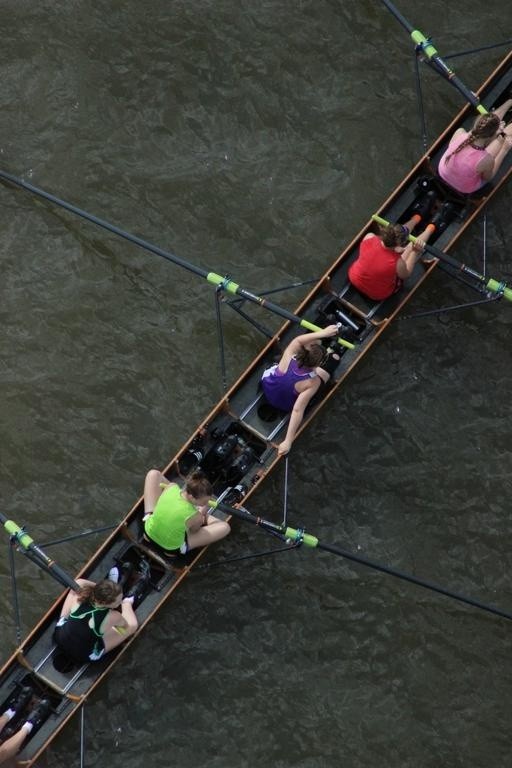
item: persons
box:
[434,98,512,193]
[347,215,436,301]
[52,566,139,673]
[0,686,49,764]
[142,467,231,559]
[259,324,341,456]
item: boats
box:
[0,38,512,768]
